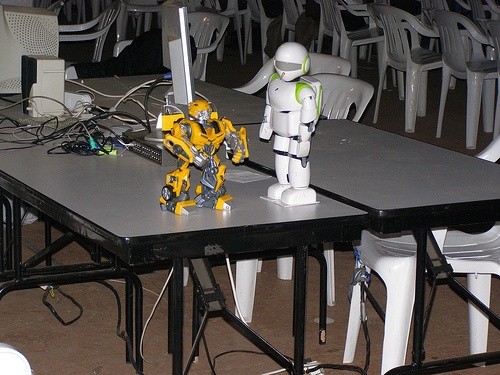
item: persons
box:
[258,41,322,205]
[157,100,249,216]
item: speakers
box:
[21,55,66,117]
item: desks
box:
[0,74,500,375]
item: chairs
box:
[46,0,500,375]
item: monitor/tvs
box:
[0,2,59,95]
[144,4,193,142]
